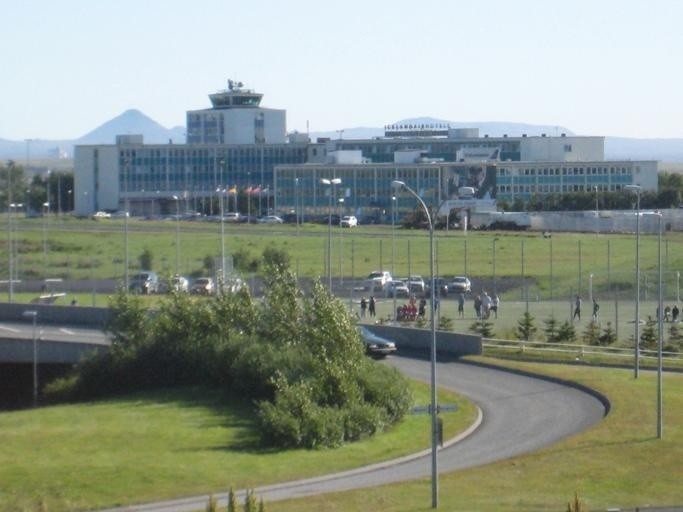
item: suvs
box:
[121,268,159,295]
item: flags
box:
[215,184,270,195]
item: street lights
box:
[21,310,42,398]
[123,210,132,292]
[10,203,23,281]
[318,177,342,296]
[171,195,181,273]
[387,179,475,509]
[620,183,644,380]
[491,237,501,294]
[642,211,663,438]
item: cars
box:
[356,323,400,360]
[364,270,474,298]
[90,207,361,229]
[190,277,215,296]
[220,276,249,297]
[159,274,190,296]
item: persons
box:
[358,291,501,323]
[663,305,671,322]
[672,305,680,320]
[574,296,581,321]
[592,299,600,322]
